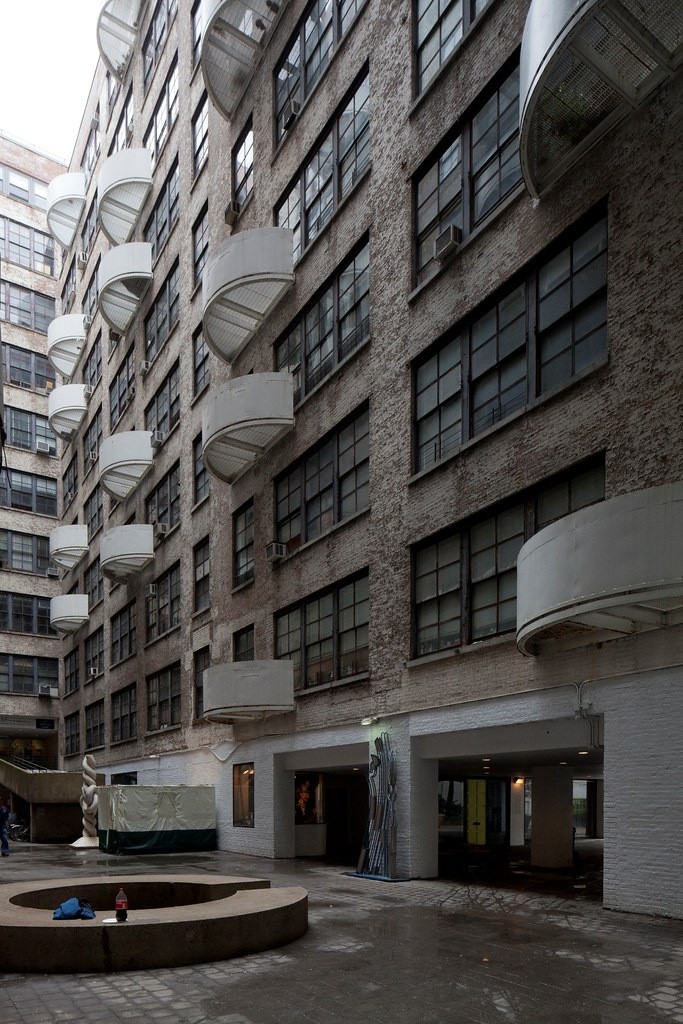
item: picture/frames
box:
[139,361,150,376]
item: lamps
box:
[361,717,378,725]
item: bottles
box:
[115,887,127,922]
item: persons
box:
[0,797,9,857]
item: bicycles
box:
[9,821,31,843]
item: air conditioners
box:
[434,223,462,261]
[153,523,167,537]
[46,568,58,576]
[83,315,91,329]
[90,111,99,130]
[39,685,51,694]
[145,584,157,597]
[265,543,286,561]
[225,201,240,225]
[78,252,87,270]
[88,667,98,676]
[37,441,49,453]
[283,99,300,131]
[151,430,165,448]
[88,452,96,463]
[83,386,89,398]
